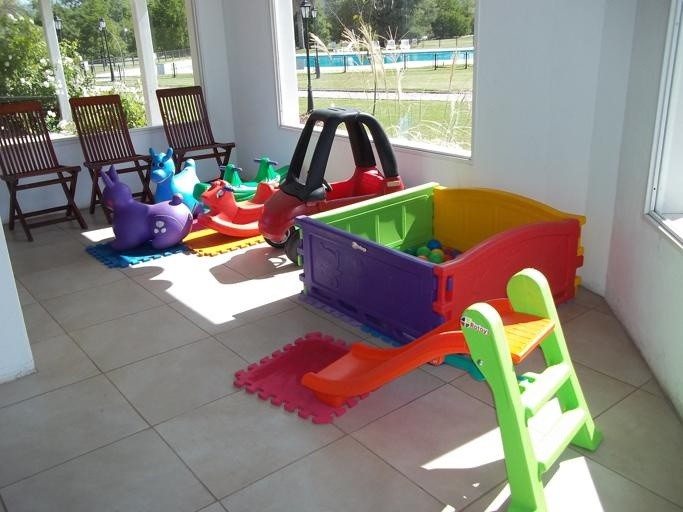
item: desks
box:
[54,16,62,43]
[99,19,114,81]
[300,0,313,113]
[312,6,320,79]
[99,25,107,65]
[124,27,134,64]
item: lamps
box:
[1,86,236,241]
